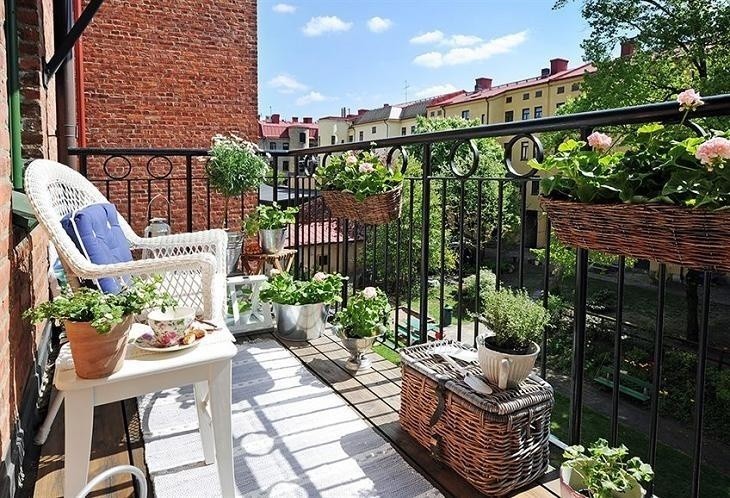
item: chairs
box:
[23,158,229,447]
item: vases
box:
[341,331,376,370]
[272,303,326,341]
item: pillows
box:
[61,202,134,294]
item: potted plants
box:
[21,273,178,379]
[560,439,654,498]
[204,132,271,276]
[242,200,299,254]
[465,280,551,389]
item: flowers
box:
[258,268,344,306]
[334,286,392,337]
[137,337,446,498]
[312,142,403,206]
[527,88,730,211]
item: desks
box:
[54,323,237,498]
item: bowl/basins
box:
[148,306,195,338]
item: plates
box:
[136,329,208,352]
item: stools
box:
[241,249,298,275]
